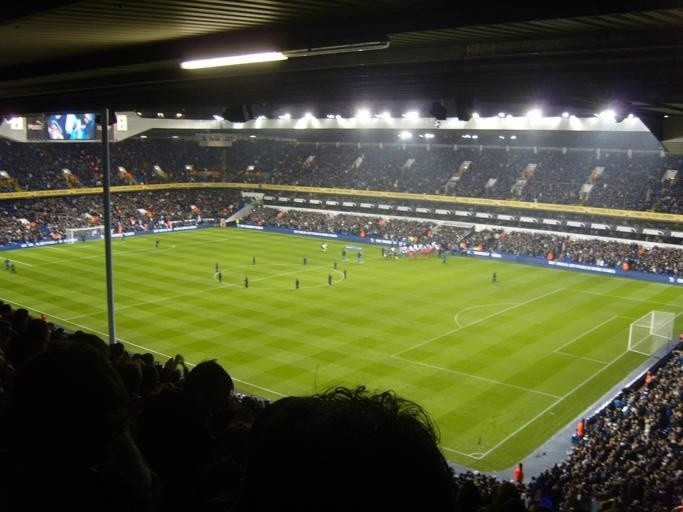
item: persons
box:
[526,326,681,512]
[344,268,347,279]
[296,278,299,290]
[243,276,248,288]
[491,272,498,282]
[217,270,222,284]
[328,272,331,286]
